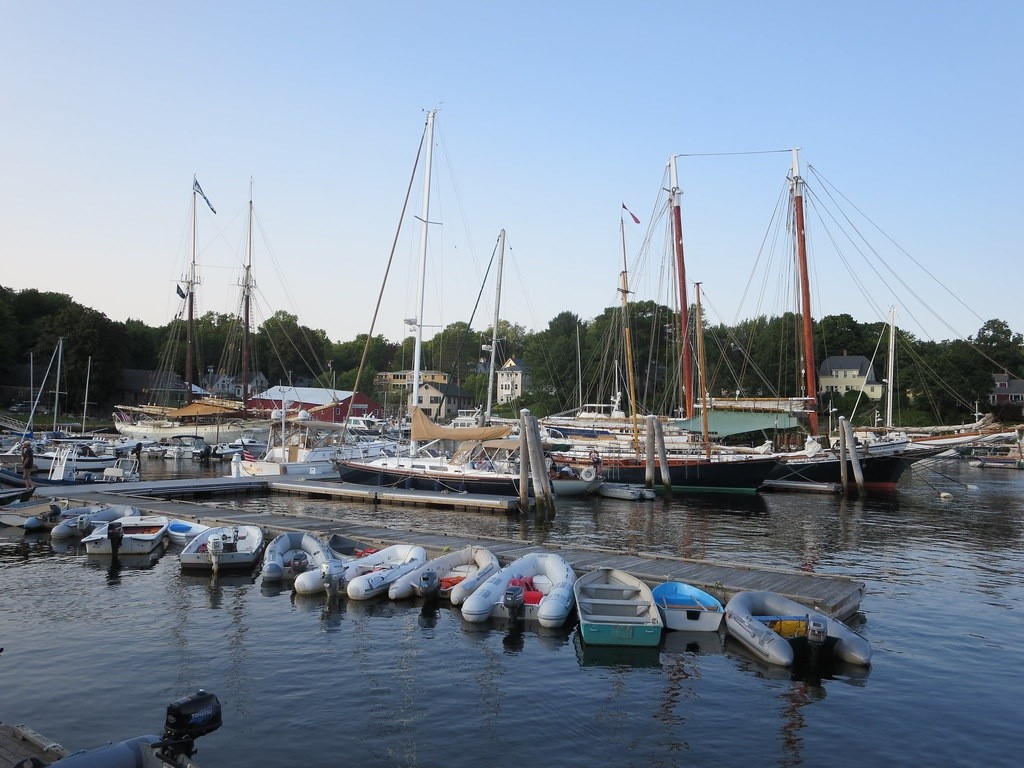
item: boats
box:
[167,517,211,545]
[326,532,380,565]
[179,525,266,572]
[573,566,665,647]
[260,531,331,583]
[80,514,171,555]
[389,545,502,606]
[48,504,142,539]
[23,505,104,533]
[0,499,70,527]
[724,591,871,677]
[461,553,577,629]
[291,544,427,602]
[651,581,725,631]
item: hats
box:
[23,441,31,444]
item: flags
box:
[242,441,255,461]
[623,203,640,223]
[193,175,217,214]
[177,285,186,299]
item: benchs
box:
[580,583,641,598]
[664,604,718,610]
[583,599,652,617]
[585,615,651,624]
[360,563,390,570]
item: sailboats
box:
[0,87,1024,510]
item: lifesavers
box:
[589,450,599,460]
[582,468,595,482]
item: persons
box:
[506,449,514,463]
[562,460,573,478]
[472,457,487,469]
[512,424,519,435]
[543,451,553,478]
[22,444,34,489]
